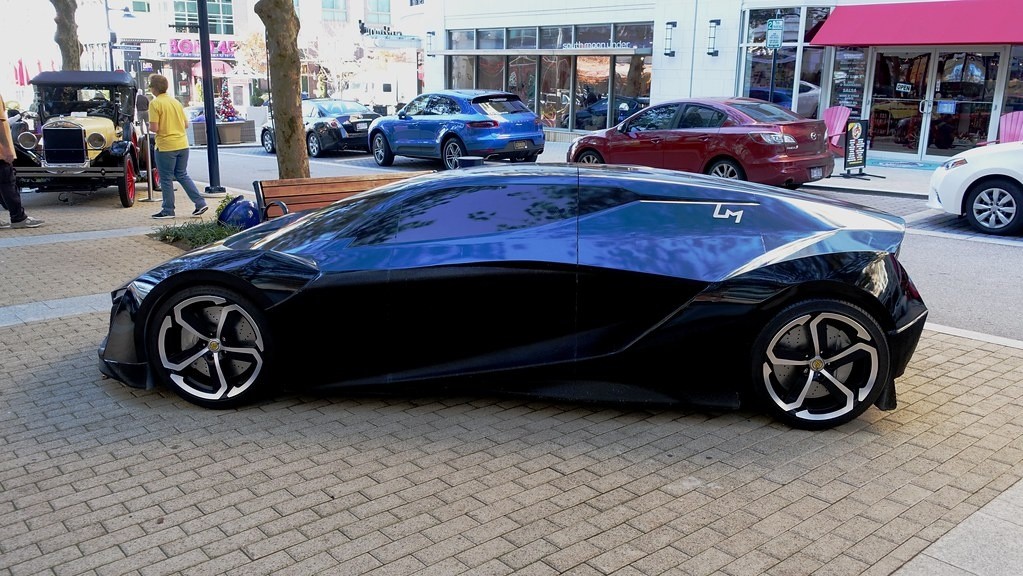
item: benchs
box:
[252,169,439,223]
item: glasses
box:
[148,85,153,88]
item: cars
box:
[260,99,386,158]
[896,109,988,149]
[368,89,546,172]
[743,85,793,111]
[561,95,651,130]
[925,140,1023,237]
[796,81,822,121]
[4,68,162,207]
[566,97,836,189]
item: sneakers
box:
[192,204,209,216]
[0,219,11,229]
[151,211,175,219]
[10,216,45,229]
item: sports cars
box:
[97,160,926,430]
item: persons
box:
[136,89,152,139]
[524,73,536,114]
[574,82,599,110]
[189,108,205,123]
[0,91,48,230]
[145,70,211,221]
